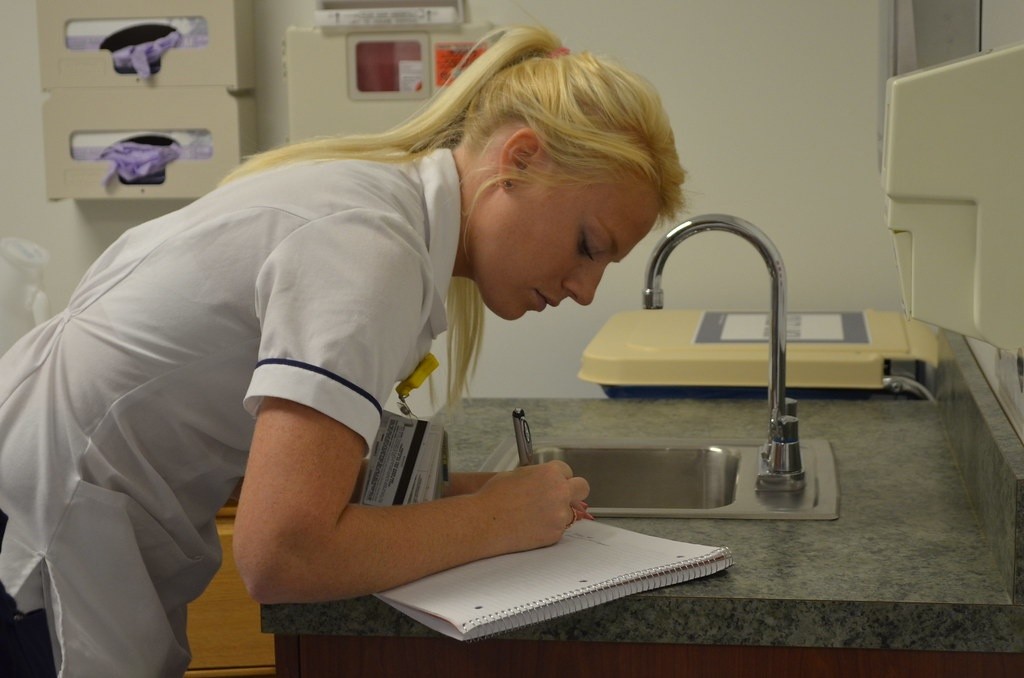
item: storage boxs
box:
[35,0,259,205]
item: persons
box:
[0,23,683,678]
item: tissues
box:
[43,91,257,201]
[36,5,258,92]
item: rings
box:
[565,507,577,528]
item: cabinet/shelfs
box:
[180,476,275,678]
[270,631,1022,678]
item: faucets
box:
[643,213,805,491]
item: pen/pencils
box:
[512,406,534,466]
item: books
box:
[372,520,734,642]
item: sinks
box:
[478,400,838,518]
